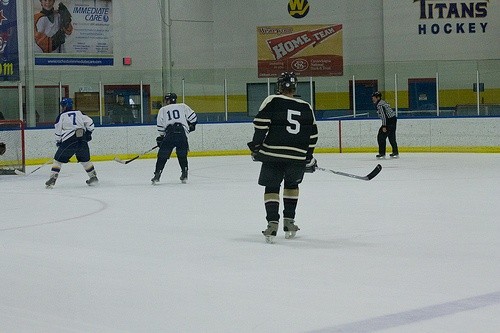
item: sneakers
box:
[283,219,300,239]
[180,172,187,184]
[151,173,160,185]
[390,152,398,159]
[45,177,56,189]
[86,177,99,185]
[262,220,279,243]
[376,152,386,160]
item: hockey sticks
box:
[15,157,54,177]
[113,145,158,165]
[314,163,384,182]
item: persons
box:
[247,72,319,243]
[45,97,98,188]
[110,93,136,125]
[34,0,73,52]
[371,91,399,160]
[151,93,198,185]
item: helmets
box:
[372,92,381,97]
[165,92,177,104]
[274,71,297,95]
[60,97,73,109]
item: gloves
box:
[83,128,92,141]
[187,120,197,131]
[247,141,263,161]
[56,140,62,146]
[305,158,318,173]
[156,137,167,147]
